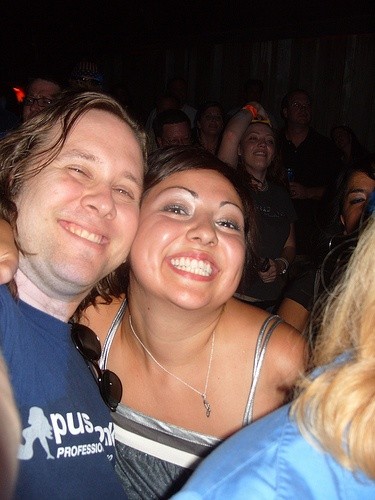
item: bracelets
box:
[243,104,258,120]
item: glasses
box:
[71,323,123,412]
[79,77,99,84]
[294,104,309,111]
[24,97,54,107]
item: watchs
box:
[273,256,289,276]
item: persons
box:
[1,64,374,499]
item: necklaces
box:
[248,172,264,190]
[128,311,215,418]
